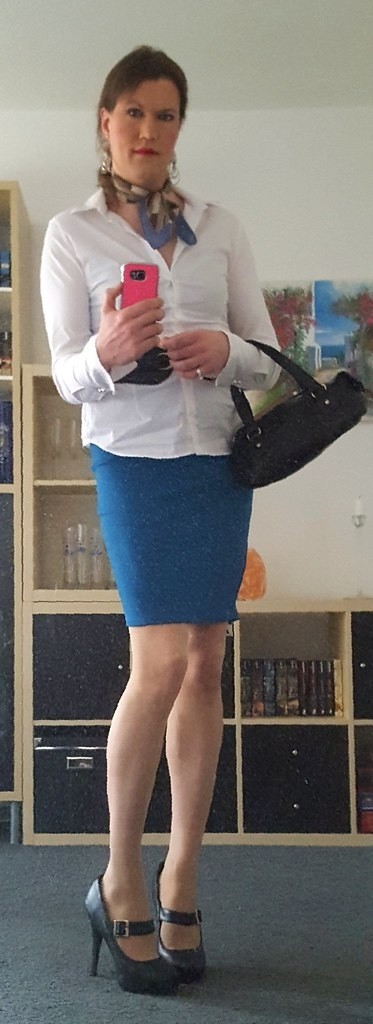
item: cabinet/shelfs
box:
[20,363,373,846]
[1,181,32,844]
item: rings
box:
[197,369,203,382]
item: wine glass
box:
[44,416,95,480]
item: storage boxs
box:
[357,791,373,833]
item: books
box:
[236,656,342,719]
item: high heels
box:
[84,875,180,995]
[154,858,208,984]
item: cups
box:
[60,522,118,592]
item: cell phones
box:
[121,264,158,313]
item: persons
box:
[39,46,282,996]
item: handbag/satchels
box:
[230,334,366,491]
